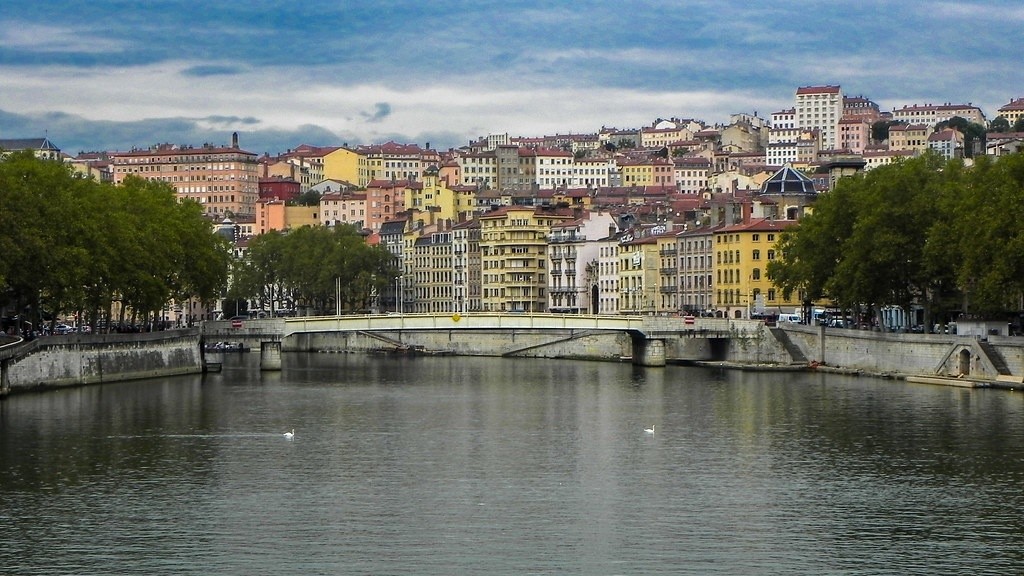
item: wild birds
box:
[283,429,295,436]
[644,425,656,432]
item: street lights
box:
[529,276,533,316]
[519,277,523,311]
[653,282,657,317]
[395,276,403,315]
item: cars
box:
[933,323,950,334]
[42,321,92,335]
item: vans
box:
[778,313,805,325]
[795,306,853,328]
